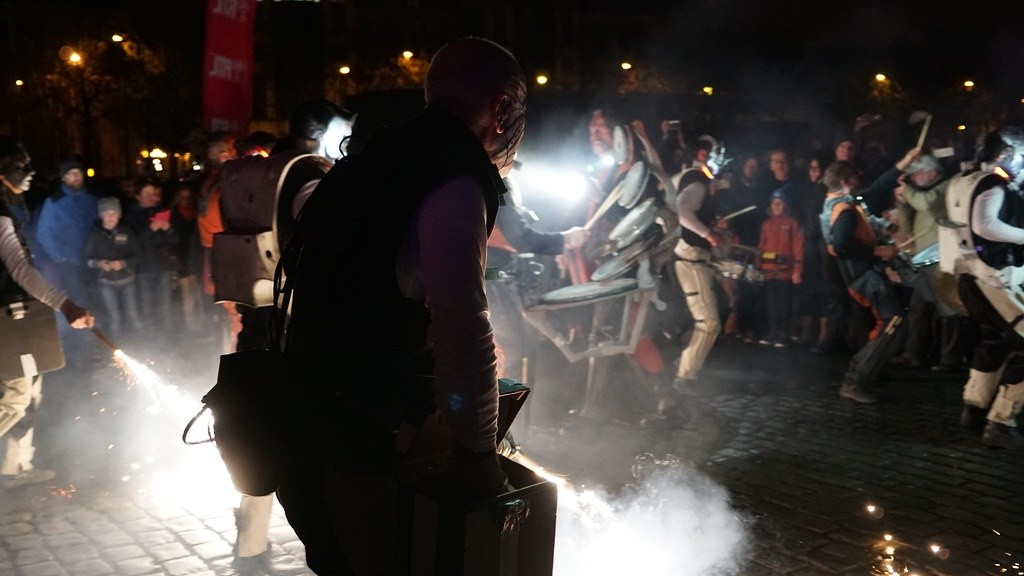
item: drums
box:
[908,242,966,318]
[711,242,763,282]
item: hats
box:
[98,197,122,215]
[771,183,793,206]
[59,160,84,180]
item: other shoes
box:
[0,467,56,489]
[736,329,824,353]
[840,382,881,405]
[673,374,718,397]
[960,406,988,428]
[982,420,1024,452]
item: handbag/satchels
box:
[181,350,301,497]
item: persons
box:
[0,50,1024,488]
[274,37,529,576]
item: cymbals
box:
[538,125,661,306]
